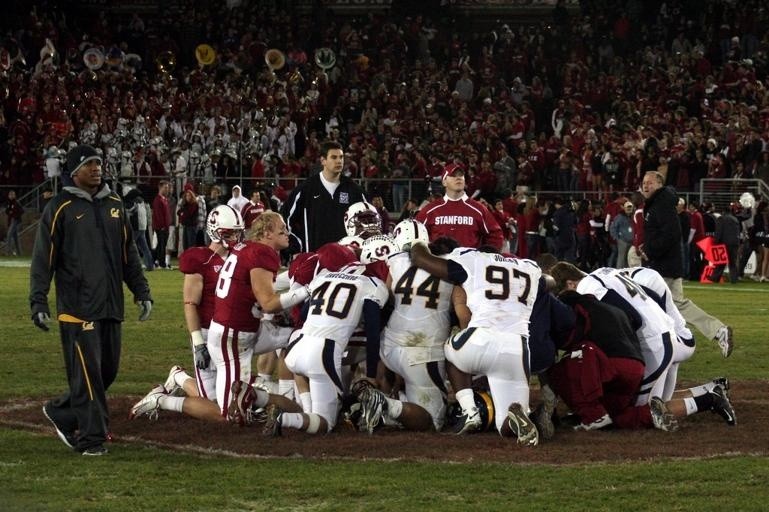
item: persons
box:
[415,161,505,256]
[635,168,734,364]
[29,146,156,460]
[127,199,740,450]
[278,143,366,265]
[1,1,768,285]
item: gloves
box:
[137,301,152,321]
[32,312,49,329]
[194,344,210,369]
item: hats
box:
[442,163,464,179]
[62,144,100,176]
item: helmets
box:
[343,379,382,432]
[206,204,245,249]
[338,202,429,264]
[445,390,493,431]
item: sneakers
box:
[715,327,732,358]
[42,403,77,447]
[362,388,384,434]
[164,366,183,396]
[649,396,681,432]
[454,407,482,435]
[709,377,736,424]
[227,377,284,439]
[509,404,613,448]
[81,444,107,456]
[128,384,166,421]
